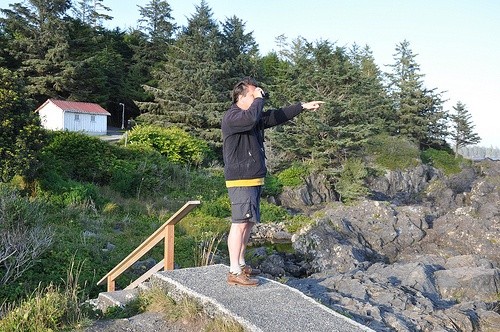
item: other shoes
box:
[227,271,260,287]
[240,265,261,277]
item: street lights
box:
[119,103,125,130]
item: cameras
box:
[261,92,269,98]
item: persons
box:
[220,80,327,286]
